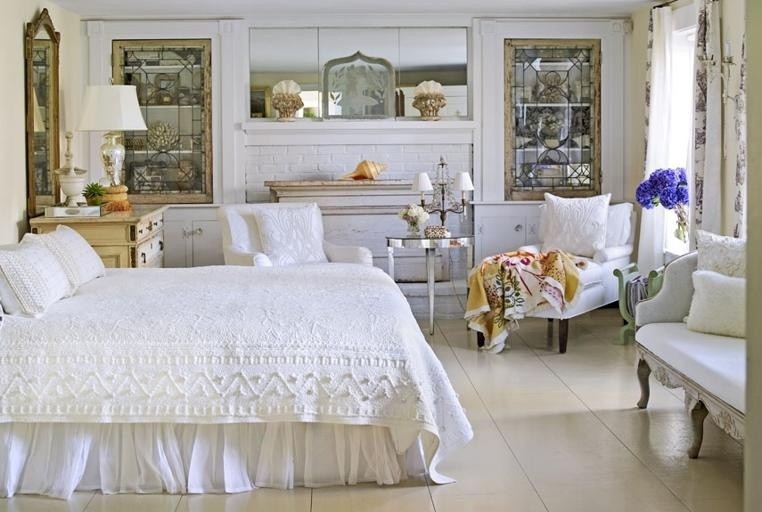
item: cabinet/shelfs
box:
[28,203,170,268]
[112,39,213,205]
[164,207,224,268]
[504,38,602,199]
[471,205,541,267]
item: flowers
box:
[636,164,689,243]
[399,203,429,224]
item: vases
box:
[406,222,420,238]
[675,212,690,243]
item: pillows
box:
[686,229,748,338]
[252,204,329,264]
[0,224,106,319]
[539,192,634,257]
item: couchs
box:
[635,250,747,459]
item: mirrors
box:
[26,7,61,217]
[243,26,472,122]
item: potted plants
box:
[80,183,106,206]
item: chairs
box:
[466,200,636,352]
[217,203,373,266]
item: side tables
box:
[385,236,475,335]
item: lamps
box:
[412,152,475,238]
[32,87,46,133]
[73,84,149,187]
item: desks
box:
[263,180,453,284]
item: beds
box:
[0,262,473,498]
